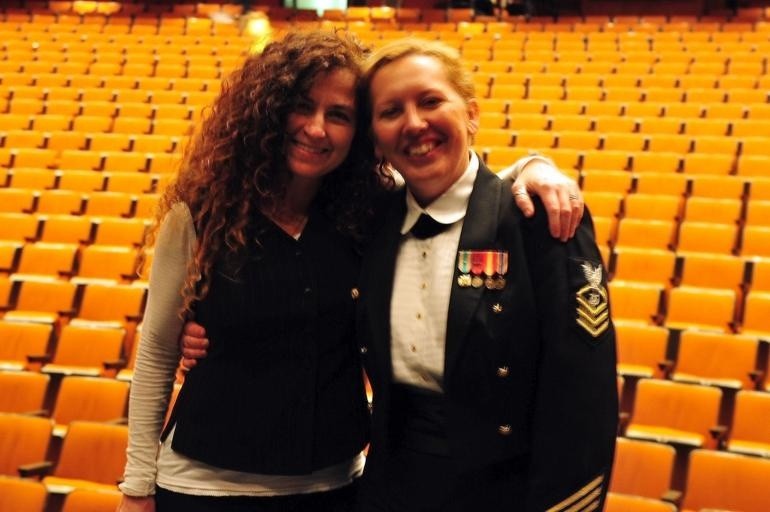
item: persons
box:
[120,32,584,510]
[179,37,617,510]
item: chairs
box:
[0,0,770,511]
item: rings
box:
[569,194,580,202]
[514,192,527,197]
